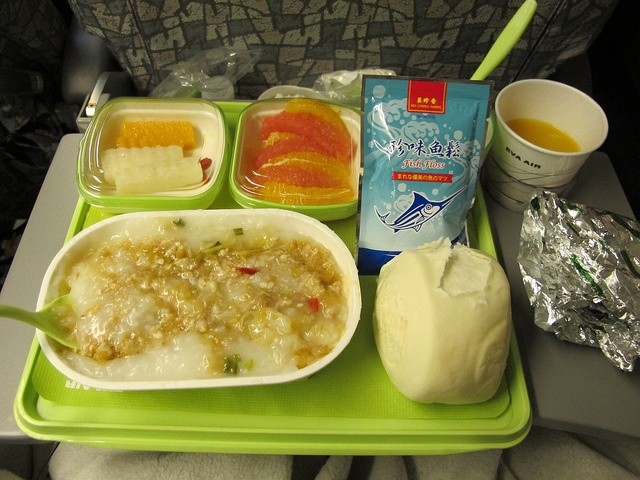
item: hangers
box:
[0,294,80,350]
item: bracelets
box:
[34,207,363,392]
[483,78,610,190]
